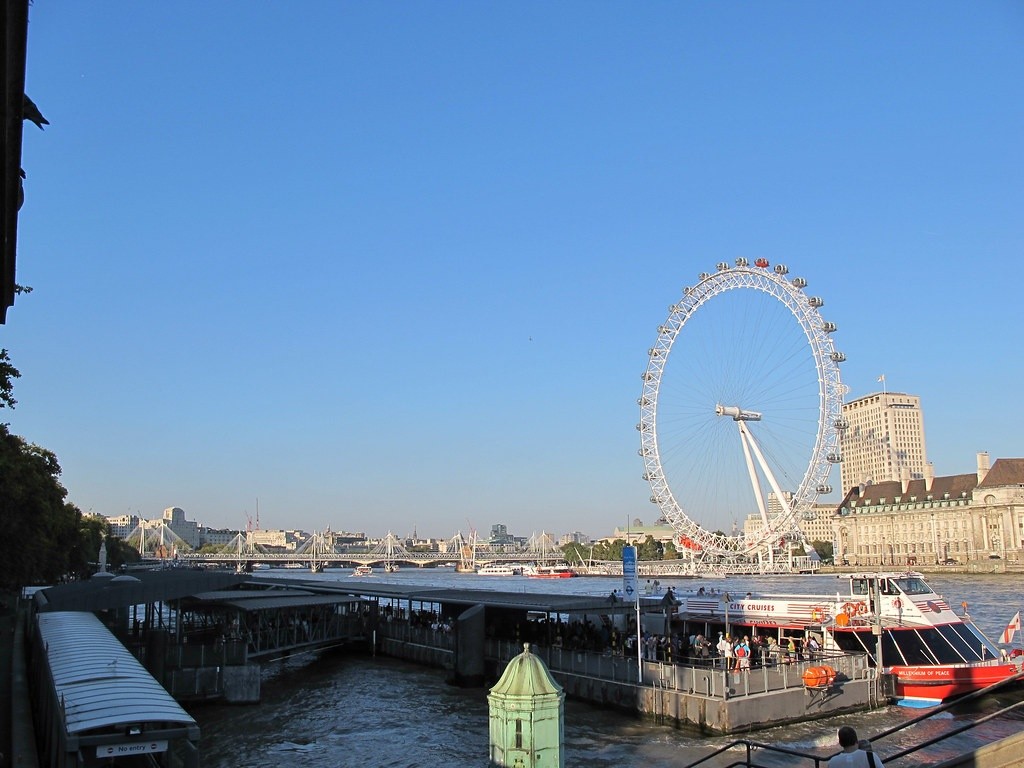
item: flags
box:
[998,611,1021,644]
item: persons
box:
[214,579,823,682]
[828,726,885,768]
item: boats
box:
[478,561,577,578]
[568,572,1024,700]
[355,566,372,576]
[252,563,270,570]
[278,563,305,569]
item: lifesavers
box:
[843,603,857,617]
[813,608,825,622]
[855,601,868,616]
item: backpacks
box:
[736,644,747,658]
